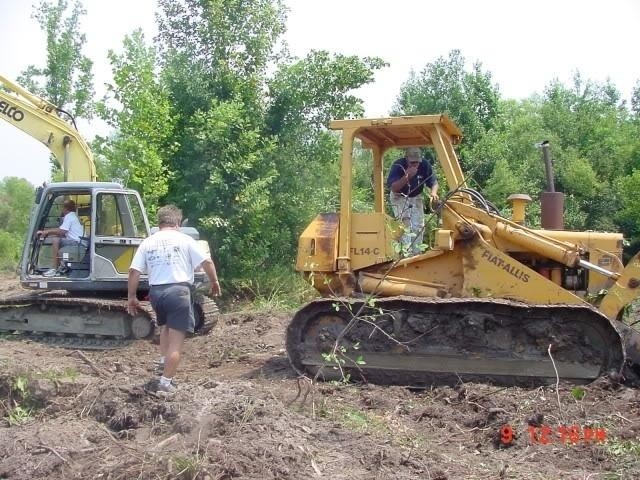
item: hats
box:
[407,147,422,163]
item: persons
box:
[387,146,440,257]
[36,199,85,277]
[127,204,221,399]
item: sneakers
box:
[158,363,165,375]
[157,383,177,393]
[43,268,60,277]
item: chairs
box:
[57,219,90,279]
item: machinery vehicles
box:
[1,66,218,349]
[282,113,640,394]
